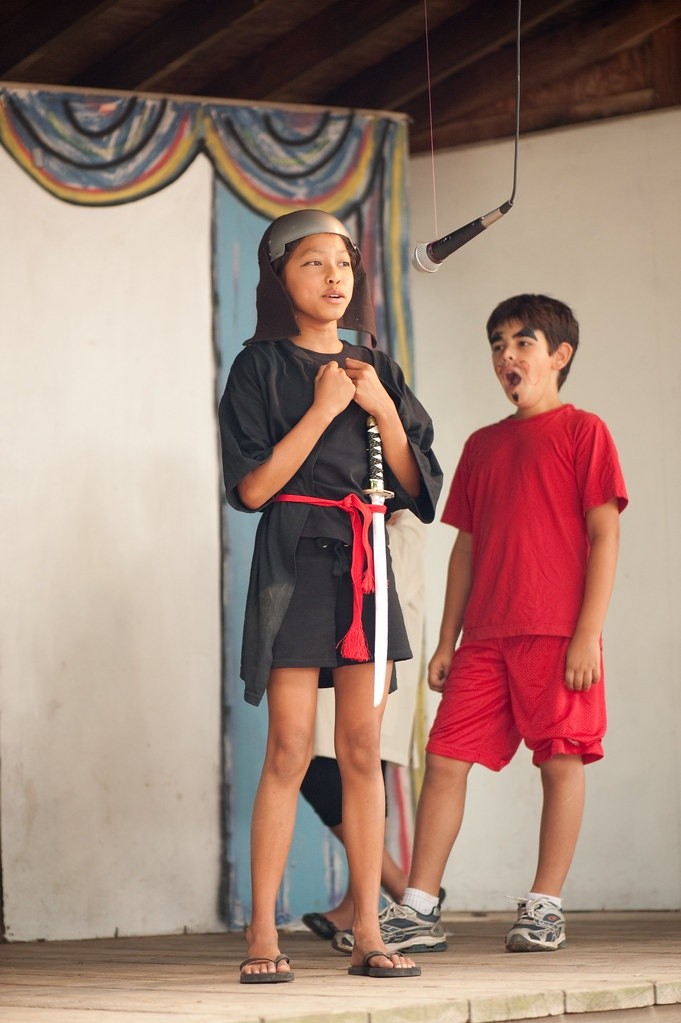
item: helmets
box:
[266,210,362,267]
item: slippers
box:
[347,950,421,977]
[239,952,294,983]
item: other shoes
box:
[301,912,352,939]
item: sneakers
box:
[330,887,448,953]
[504,895,568,952]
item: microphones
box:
[411,200,513,273]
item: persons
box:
[218,210,444,982]
[300,512,446,940]
[333,294,628,953]
